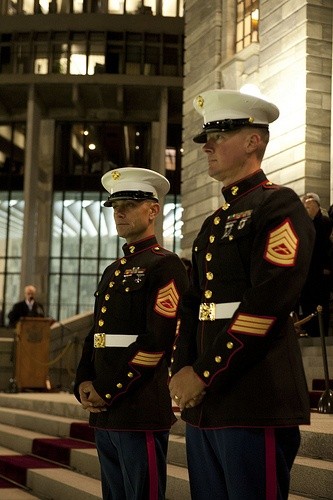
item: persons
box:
[169,91,314,500]
[8,285,45,384]
[74,167,187,500]
[303,192,333,338]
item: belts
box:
[93,332,138,348]
[198,302,244,322]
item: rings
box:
[175,395,179,400]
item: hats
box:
[193,89,280,144]
[305,193,321,206]
[101,166,170,208]
